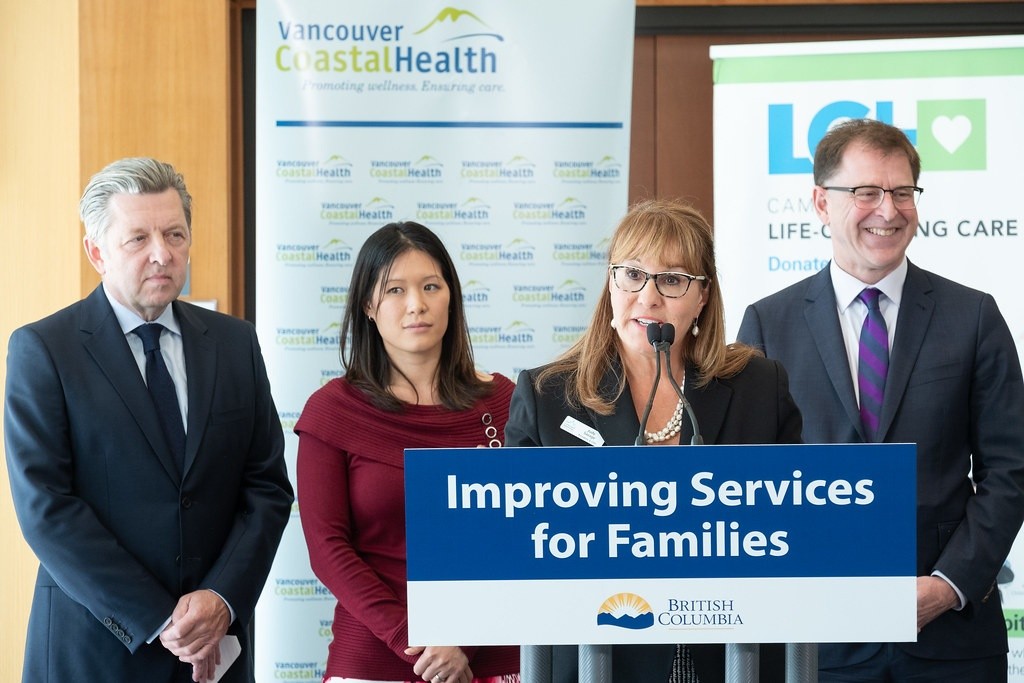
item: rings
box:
[435,674,445,682]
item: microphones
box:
[634,322,706,445]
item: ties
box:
[857,287,889,443]
[130,322,186,483]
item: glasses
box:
[607,263,710,298]
[820,185,924,210]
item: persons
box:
[503,200,804,683]
[735,120,1024,683]
[4,159,295,683]
[297,222,520,683]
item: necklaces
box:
[644,370,685,444]
